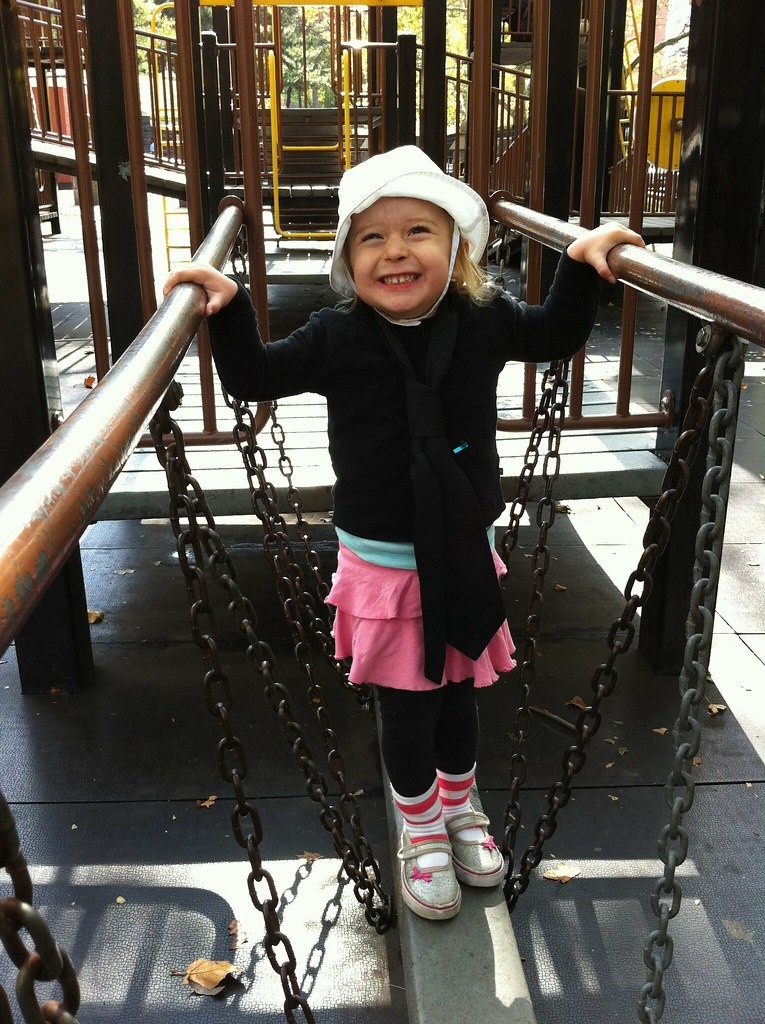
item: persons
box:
[162,143,646,920]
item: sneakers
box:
[398,824,462,919]
[445,803,505,887]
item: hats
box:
[329,145,490,326]
